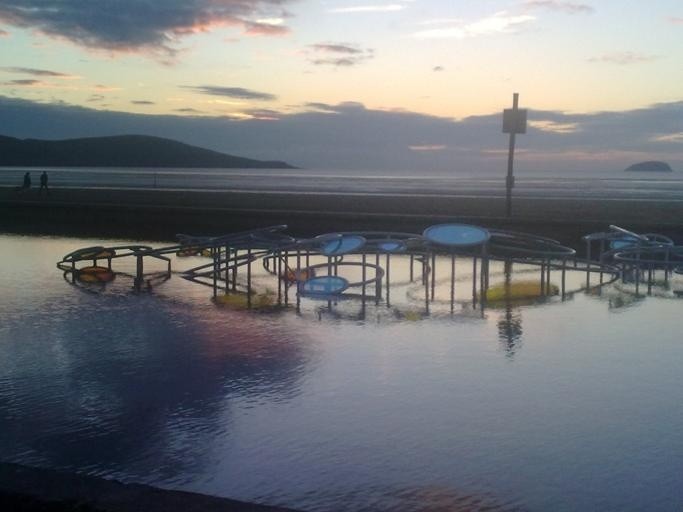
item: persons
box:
[37,171,50,195]
[15,171,32,193]
